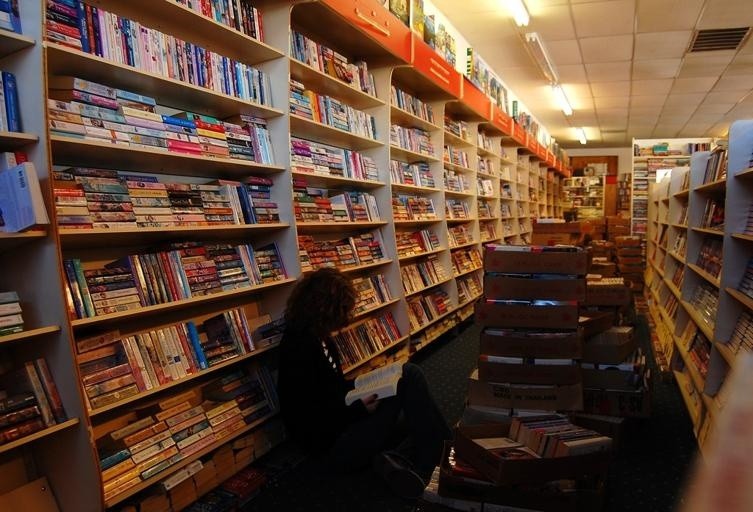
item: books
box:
[290,0,565,406]
[0,0,287,511]
[566,139,751,511]
[425,406,565,511]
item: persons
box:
[277,268,452,481]
